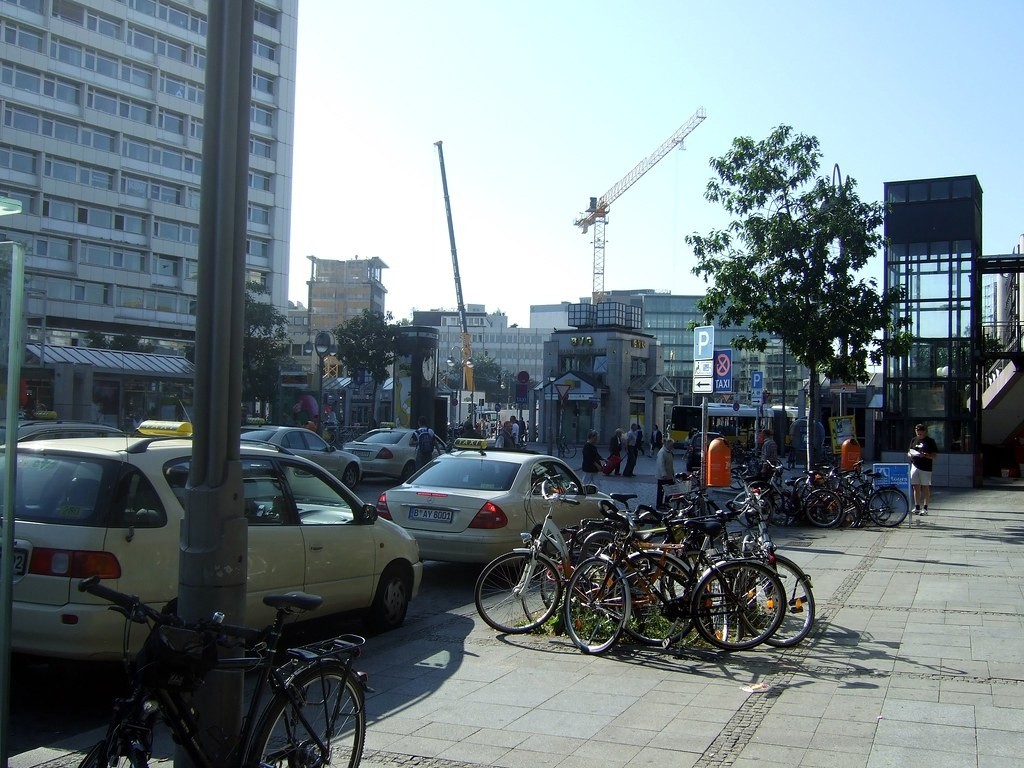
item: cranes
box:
[572,106,708,296]
[434,140,475,390]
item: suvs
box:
[0,409,424,663]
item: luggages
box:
[602,452,627,475]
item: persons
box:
[495,421,516,447]
[327,407,336,421]
[510,416,519,444]
[521,417,526,441]
[761,429,777,474]
[497,421,503,435]
[409,416,441,473]
[908,424,937,515]
[462,421,482,438]
[241,406,250,426]
[656,439,674,511]
[476,419,491,436]
[609,428,623,475]
[649,425,663,455]
[581,430,602,487]
[623,424,644,477]
[681,427,694,458]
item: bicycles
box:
[473,450,910,653]
[557,432,577,459]
[77,574,377,768]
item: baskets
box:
[661,481,691,496]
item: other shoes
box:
[622,472,634,477]
[663,502,673,509]
[657,506,669,512]
[647,454,652,458]
[632,474,637,476]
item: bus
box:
[774,406,799,451]
[670,403,775,451]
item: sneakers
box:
[919,509,928,516]
[913,509,920,515]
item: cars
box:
[241,417,362,488]
[341,421,447,485]
[378,437,614,577]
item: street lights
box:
[547,374,556,455]
[304,328,331,435]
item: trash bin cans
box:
[841,438,861,471]
[707,438,731,488]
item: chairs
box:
[71,478,99,506]
[468,463,495,485]
